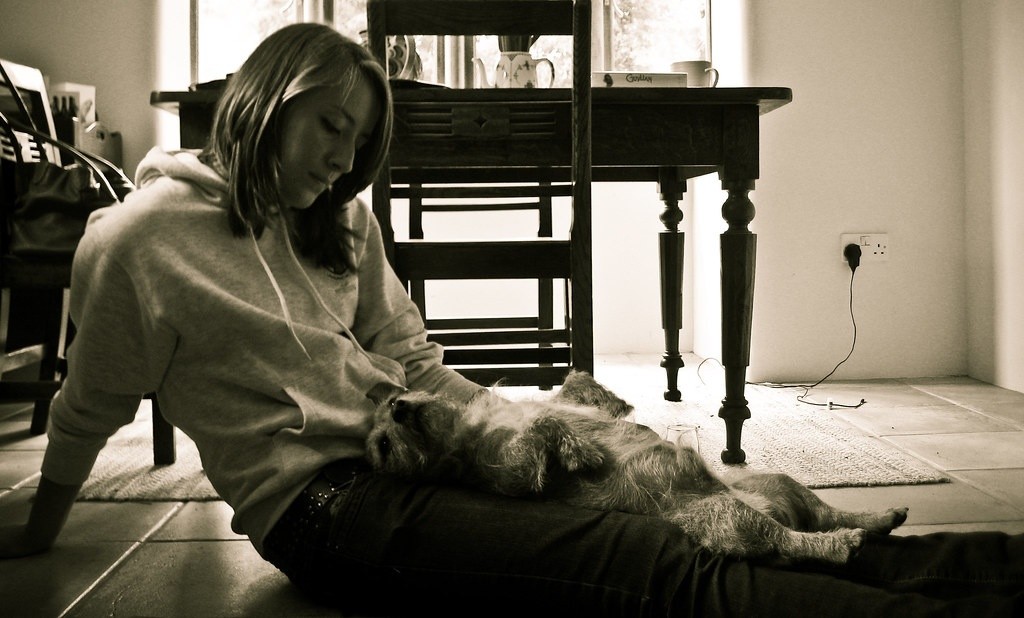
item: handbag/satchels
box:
[5,115,131,262]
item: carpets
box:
[76,380,951,503]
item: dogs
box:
[365,369,911,569]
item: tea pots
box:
[359,30,423,81]
[471,51,555,88]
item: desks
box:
[131,85,793,466]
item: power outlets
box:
[842,232,895,262]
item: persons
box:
[0,23,1024,618]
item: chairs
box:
[0,59,177,466]
[367,0,592,391]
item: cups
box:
[671,60,719,88]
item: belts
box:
[281,447,392,576]
